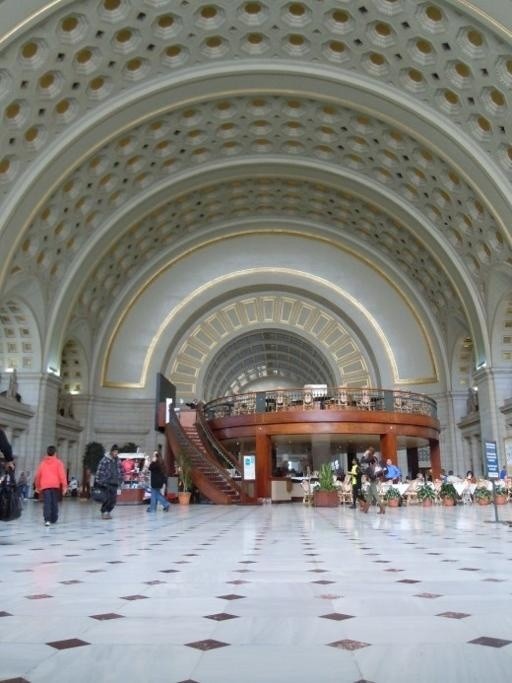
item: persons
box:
[349,458,366,510]
[69,478,80,497]
[500,467,506,479]
[342,458,480,487]
[17,470,41,502]
[146,453,171,514]
[96,444,125,520]
[360,447,385,514]
[35,445,68,526]
[0,425,15,489]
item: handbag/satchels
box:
[0,481,22,521]
[91,487,110,502]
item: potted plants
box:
[438,482,462,506]
[176,449,195,505]
[494,485,506,505]
[475,487,490,505]
[313,464,338,507]
[416,485,435,507]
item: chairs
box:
[299,479,418,507]
